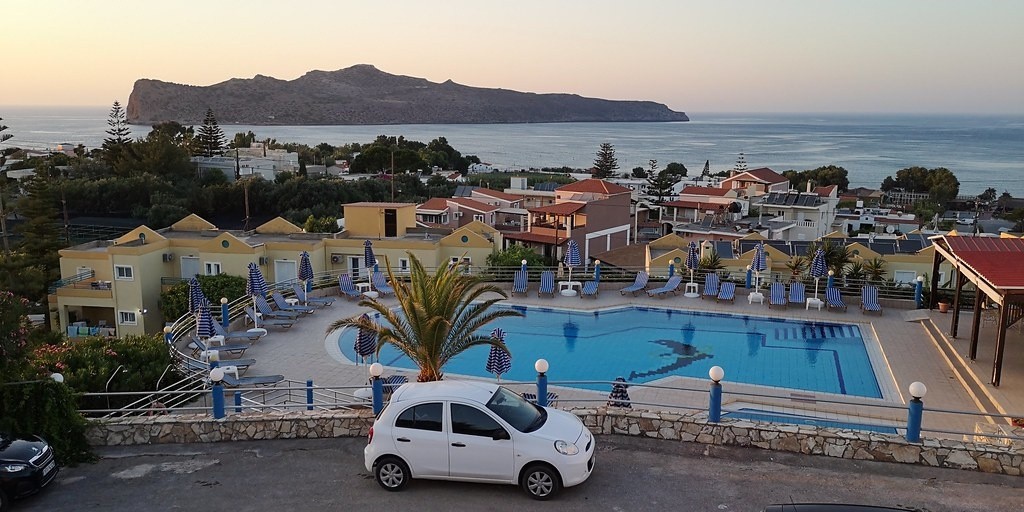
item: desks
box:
[244,313,263,322]
[685,283,699,295]
[570,281,582,291]
[749,292,764,305]
[357,283,369,292]
[207,335,226,347]
[198,349,221,362]
[285,297,299,307]
[806,298,821,311]
[219,366,239,381]
[557,281,570,293]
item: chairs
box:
[523,389,557,408]
[825,288,849,312]
[619,271,649,297]
[366,374,408,394]
[538,271,555,298]
[580,281,600,299]
[768,283,787,312]
[338,274,363,301]
[717,282,736,304]
[860,286,883,317]
[180,283,335,387]
[788,282,806,309]
[702,273,719,300]
[511,270,528,296]
[646,275,682,297]
[369,272,396,295]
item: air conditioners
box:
[163,252,174,263]
[260,256,269,264]
[332,254,344,264]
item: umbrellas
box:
[188,277,216,372]
[246,262,270,329]
[485,328,512,381]
[810,249,828,298]
[353,313,378,385]
[607,376,632,408]
[751,243,767,293]
[563,240,581,290]
[362,240,376,292]
[298,251,314,310]
[684,241,698,293]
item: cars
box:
[364,380,596,500]
[0,426,60,512]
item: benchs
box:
[73,278,96,290]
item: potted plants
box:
[938,297,951,315]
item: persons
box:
[149,393,168,416]
